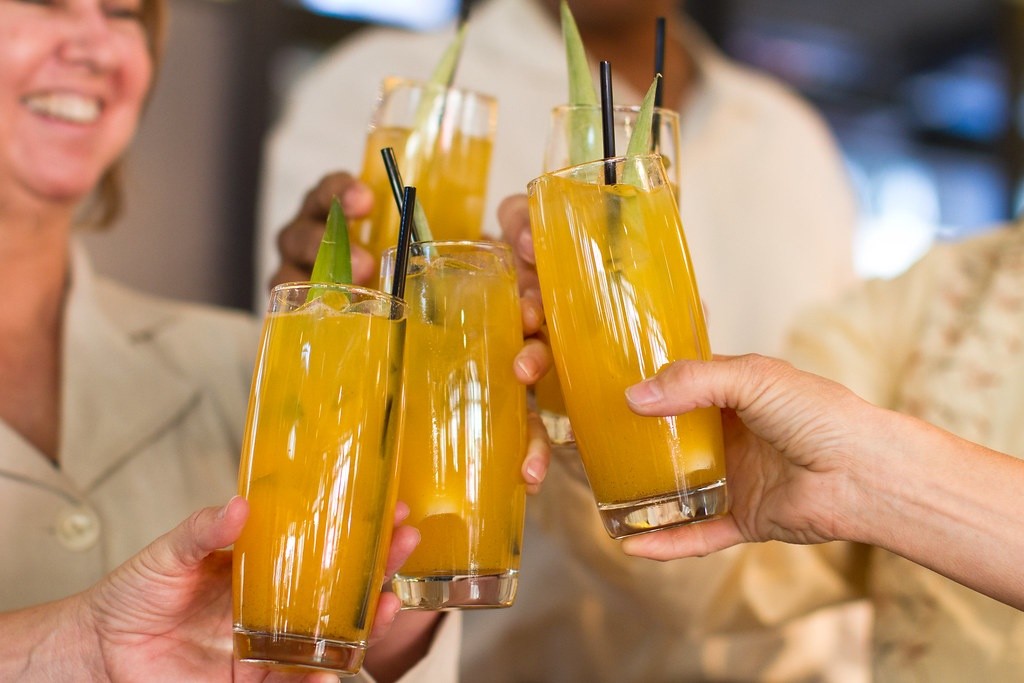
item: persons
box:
[248,1,876,683]
[1,0,551,683]
[616,351,1023,617]
[495,194,1023,682]
[2,494,424,683]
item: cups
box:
[525,153,732,540]
[231,279,410,675]
[552,103,683,211]
[361,70,502,290]
[378,239,528,611]
